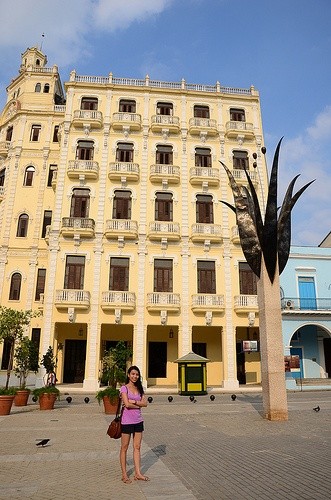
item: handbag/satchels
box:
[107,417,122,439]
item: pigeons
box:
[36,437,51,448]
[312,405,321,413]
[193,399,197,403]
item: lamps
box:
[169,328,174,338]
[79,324,84,337]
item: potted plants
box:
[32,346,62,410]
[94,339,132,414]
[14,336,34,406]
[0,305,41,415]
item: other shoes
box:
[121,475,132,484]
[134,476,150,481]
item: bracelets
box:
[133,399,137,405]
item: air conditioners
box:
[284,300,294,307]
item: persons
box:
[120,366,151,484]
